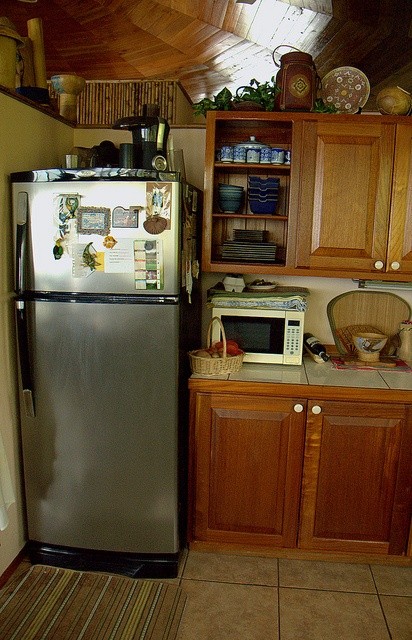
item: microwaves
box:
[209,303,305,365]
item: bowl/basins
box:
[351,331,388,362]
[218,183,243,213]
[249,176,284,214]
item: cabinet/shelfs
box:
[301,113,412,281]
[180,379,412,559]
[203,107,301,276]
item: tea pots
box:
[241,135,262,148]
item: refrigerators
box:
[8,179,189,577]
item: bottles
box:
[272,45,322,113]
[303,333,329,362]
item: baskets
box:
[187,318,245,376]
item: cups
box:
[234,145,246,163]
[248,146,260,163]
[272,147,283,165]
[286,146,291,166]
[216,143,232,164]
[261,144,271,164]
